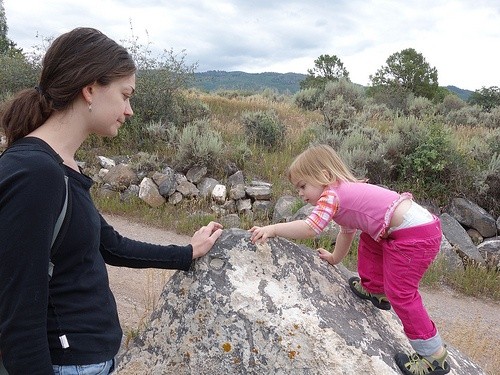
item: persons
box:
[2,28,223,375]
[249,145,450,375]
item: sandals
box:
[350,276,391,310]
[395,347,451,375]
